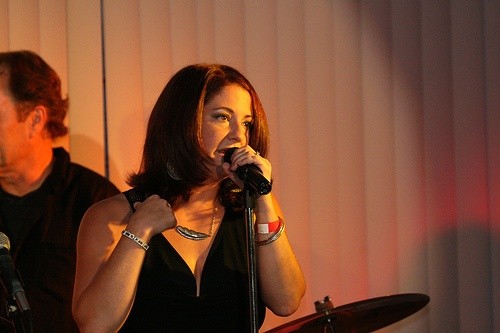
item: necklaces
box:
[176,198,220,241]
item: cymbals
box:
[261,293,431,333]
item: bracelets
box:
[121,230,149,250]
[253,217,285,247]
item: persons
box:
[71,64,306,333]
[0,50,121,333]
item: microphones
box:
[0,233,33,320]
[223,147,273,194]
